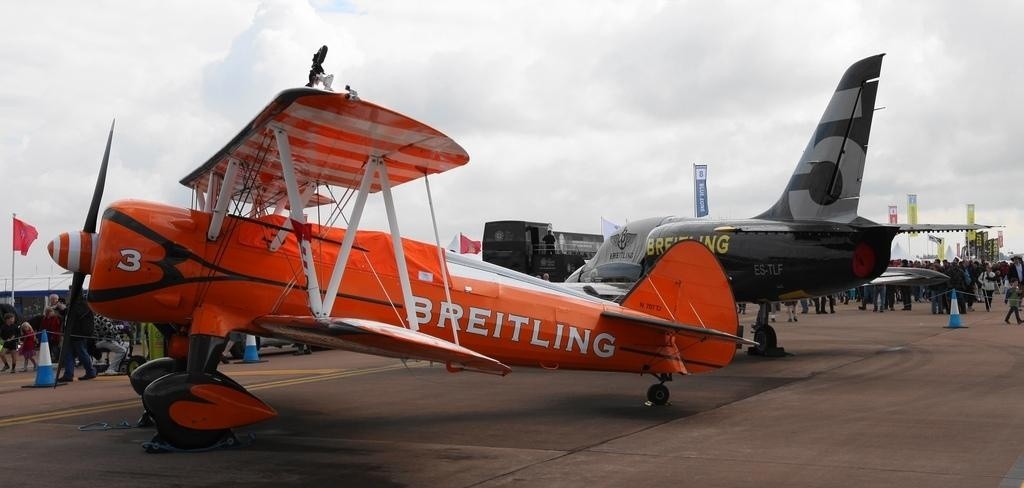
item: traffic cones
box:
[21,328,67,389]
[232,331,269,365]
[940,287,969,329]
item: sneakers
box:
[105,370,119,375]
[58,376,73,382]
[78,375,96,379]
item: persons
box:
[738,303,747,313]
[543,230,556,255]
[767,255,1023,324]
[2,286,126,381]
[543,273,551,282]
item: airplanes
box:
[48,43,761,452]
[562,53,1008,354]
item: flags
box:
[461,235,480,253]
[13,217,39,255]
